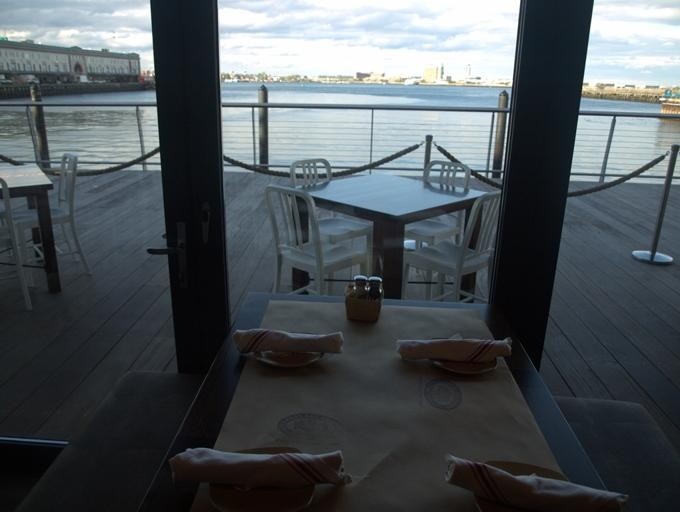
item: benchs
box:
[18,368,197,511]
[554,395,680,512]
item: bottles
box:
[350,275,383,299]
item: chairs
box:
[403,161,471,277]
[0,178,33,311]
[400,189,503,304]
[291,158,372,280]
[9,152,93,288]
[265,184,371,297]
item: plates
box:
[252,331,325,369]
[206,446,317,512]
[429,336,499,383]
[469,459,571,512]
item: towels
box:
[232,329,345,352]
[442,452,626,509]
[169,447,352,488]
[397,337,512,362]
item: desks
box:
[292,170,491,299]
[0,163,62,295]
[142,292,608,512]
